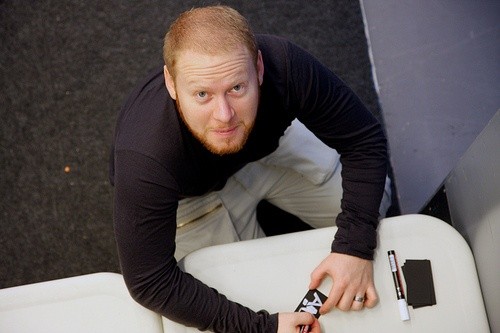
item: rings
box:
[353,296,366,302]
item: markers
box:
[387,249,411,322]
[299,311,312,333]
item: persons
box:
[108,5,391,333]
